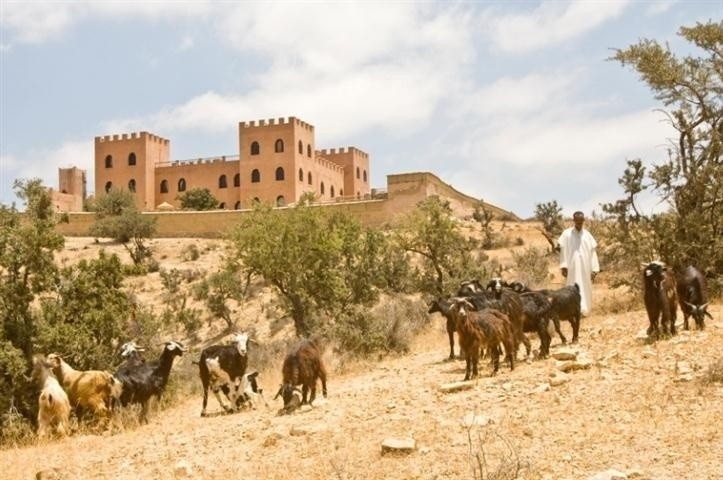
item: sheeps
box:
[198,331,327,417]
[676,265,714,331]
[21,337,188,440]
[640,260,678,337]
[426,278,580,380]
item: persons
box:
[557,211,600,318]
